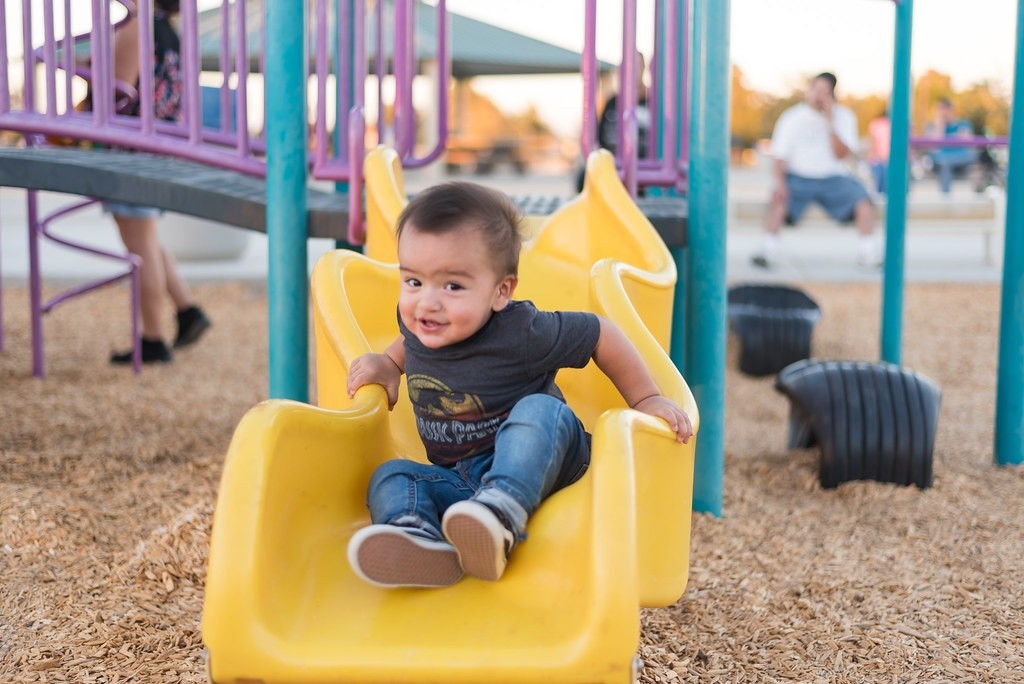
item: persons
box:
[342,181,696,590]
[577,45,651,195]
[761,72,977,232]
[50,0,214,365]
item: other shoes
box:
[752,233,773,267]
[347,501,515,587]
[174,306,209,347]
[111,337,168,362]
[855,254,881,273]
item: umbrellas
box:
[30,0,615,74]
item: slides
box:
[199,144,701,684]
